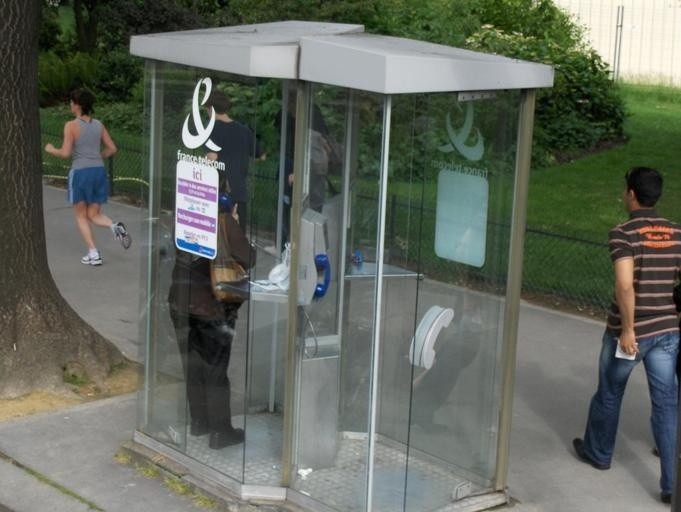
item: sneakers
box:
[189,424,206,436]
[573,439,611,468]
[208,427,244,449]
[114,223,132,249]
[81,253,103,266]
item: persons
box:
[45,90,131,265]
[274,83,341,276]
[167,170,257,450]
[573,166,681,503]
[205,91,267,236]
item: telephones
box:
[297,209,331,307]
[221,193,234,212]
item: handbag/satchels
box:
[209,252,250,303]
